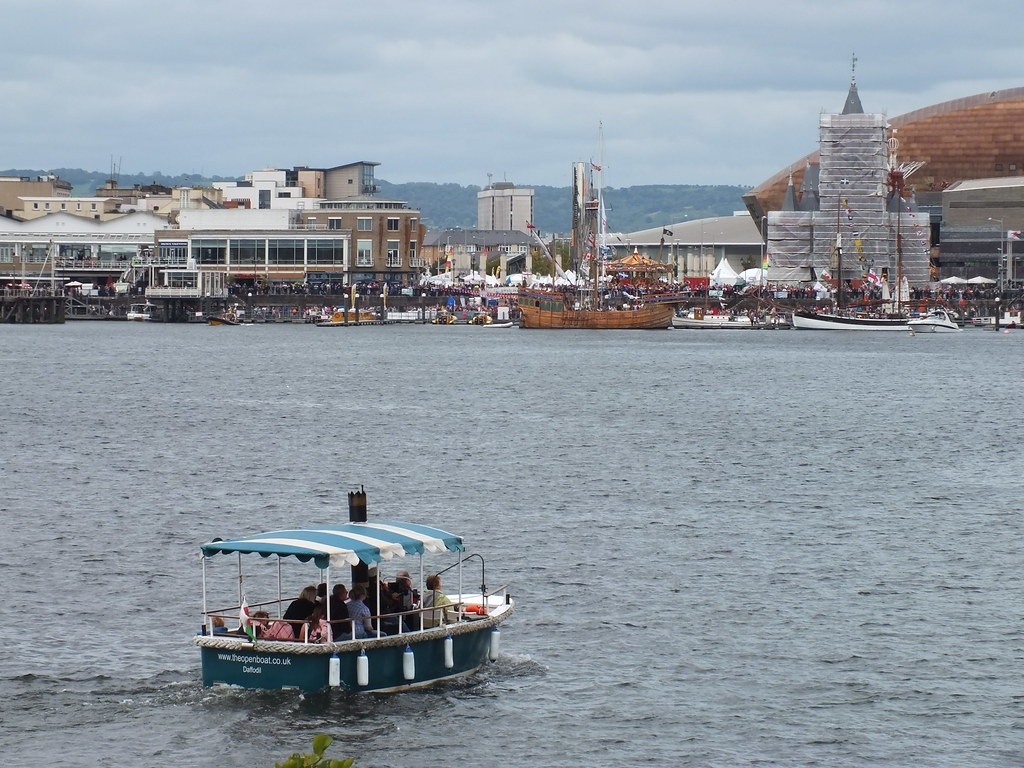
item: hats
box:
[395,572,412,581]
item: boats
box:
[191,485,515,706]
[124,120,1024,332]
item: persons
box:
[235,611,334,643]
[346,585,388,639]
[3,267,1024,335]
[196,616,228,637]
[384,570,420,612]
[364,576,410,633]
[322,584,355,641]
[281,586,322,643]
[316,582,329,606]
[417,574,457,629]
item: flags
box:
[431,153,1022,265]
